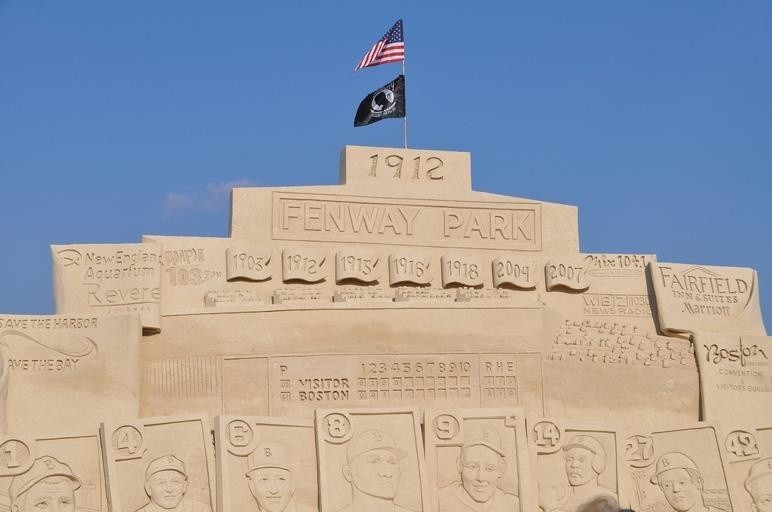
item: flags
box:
[353,17,406,74]
[353,74,406,128]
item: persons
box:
[245,439,320,511]
[134,453,214,511]
[535,433,617,511]
[435,424,521,510]
[335,428,420,511]
[648,451,730,511]
[7,452,83,511]
[743,456,772,511]
